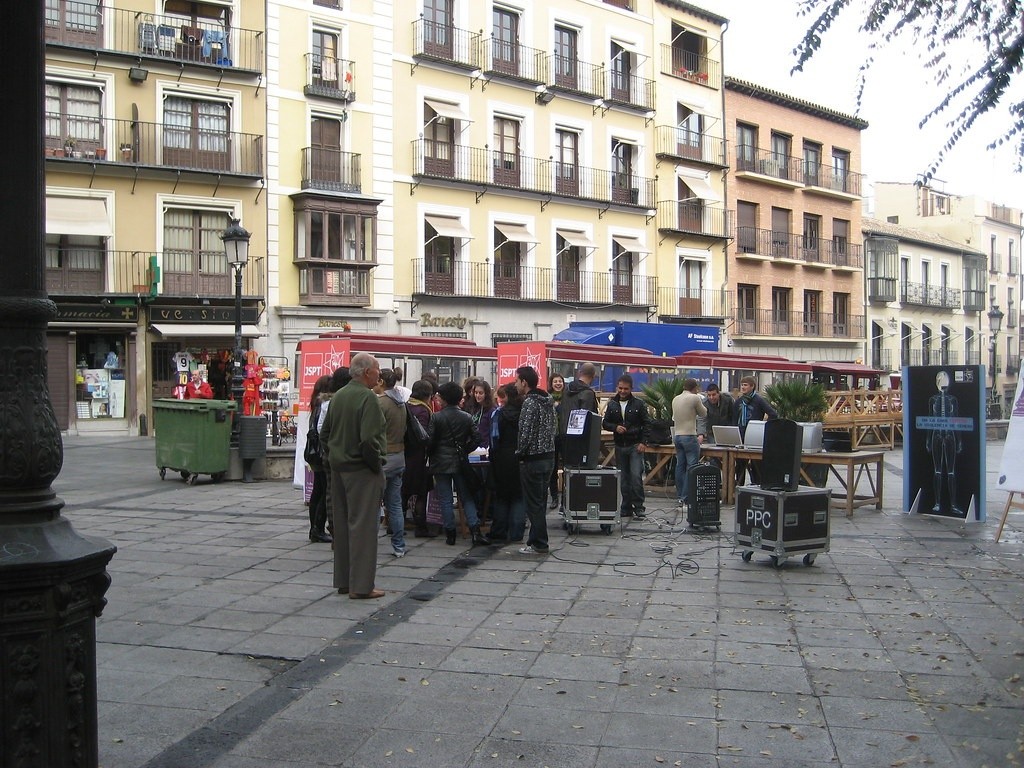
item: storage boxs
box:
[560,466,623,526]
[733,482,832,557]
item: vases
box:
[96,148,106,159]
[55,149,65,158]
[72,151,82,158]
[683,74,699,82]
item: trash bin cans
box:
[151,398,238,484]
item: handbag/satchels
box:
[404,402,434,451]
[304,428,322,465]
[457,457,483,496]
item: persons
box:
[82,369,101,392]
[697,376,778,487]
[304,362,599,555]
[184,370,213,399]
[603,374,651,516]
[319,351,387,600]
[672,378,707,513]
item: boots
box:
[445,527,456,545]
[413,511,439,537]
[470,525,491,544]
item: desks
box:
[728,448,886,519]
[279,414,298,447]
[604,442,732,506]
[302,461,524,540]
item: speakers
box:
[760,419,804,492]
[565,410,602,470]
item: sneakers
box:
[518,546,549,555]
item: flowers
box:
[674,66,708,81]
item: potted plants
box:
[61,135,78,157]
[746,377,830,488]
[120,143,131,159]
[45,142,56,157]
[85,143,97,159]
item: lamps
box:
[128,66,149,84]
[539,93,556,105]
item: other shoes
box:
[486,533,507,538]
[550,495,559,509]
[393,548,405,558]
[349,589,385,599]
[620,508,632,517]
[636,506,646,520]
[338,587,349,593]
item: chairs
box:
[137,23,157,55]
[157,27,175,58]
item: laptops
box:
[660,427,676,447]
[712,426,744,448]
[822,431,858,453]
